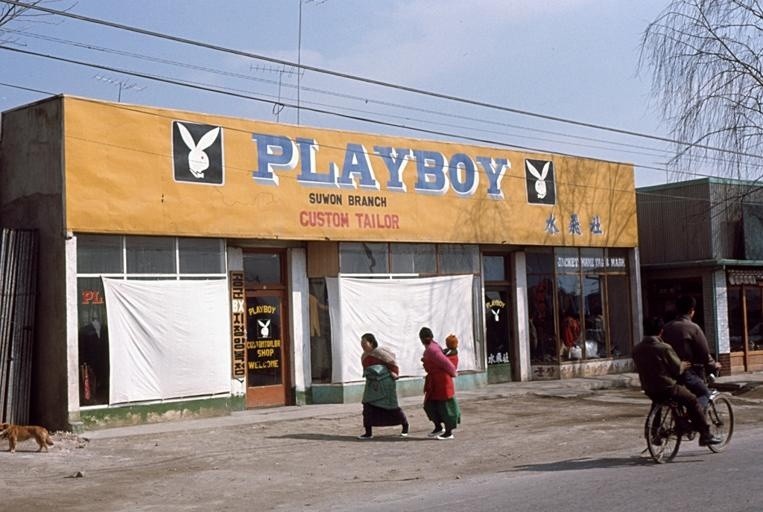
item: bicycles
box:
[639,360,736,464]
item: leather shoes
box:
[700,435,721,446]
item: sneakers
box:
[358,432,374,440]
[429,426,454,440]
[400,423,410,437]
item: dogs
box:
[0,423,54,453]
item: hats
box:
[445,334,459,350]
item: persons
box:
[442,334,458,370]
[629,312,722,448]
[660,293,723,435]
[79,312,107,394]
[418,325,459,441]
[356,332,409,439]
